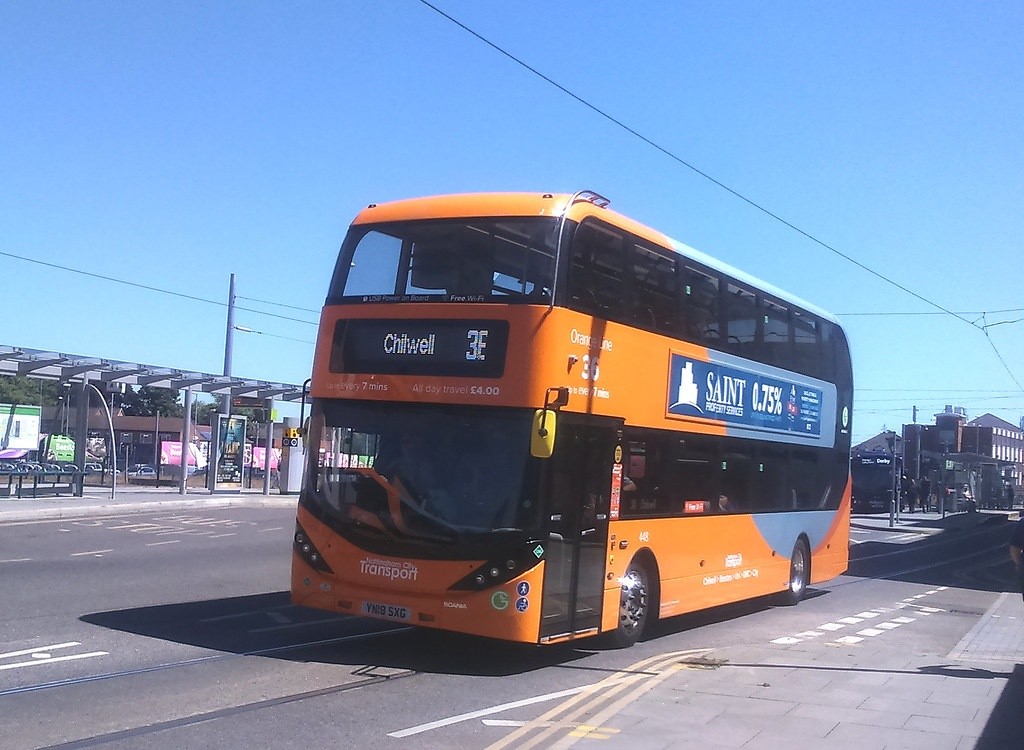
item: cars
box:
[128,466,156,477]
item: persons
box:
[584,476,637,516]
[961,484,976,513]
[1009,517,1024,602]
[907,478,921,514]
[385,432,430,501]
[1007,485,1014,511]
[935,481,947,511]
[918,476,930,513]
[718,495,728,510]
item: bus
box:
[287,188,855,650]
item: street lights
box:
[192,392,199,444]
[62,383,71,436]
[58,395,64,434]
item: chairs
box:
[524,286,779,366]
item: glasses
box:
[401,439,415,444]
